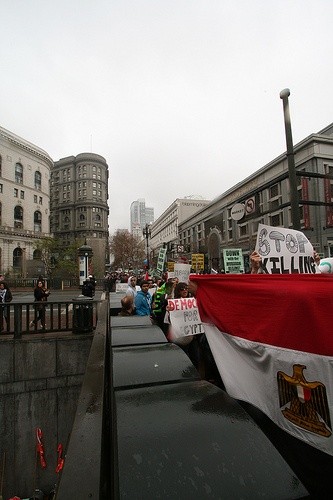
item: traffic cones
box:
[145,271,149,281]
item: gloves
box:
[95,281,97,283]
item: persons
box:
[134,281,153,318]
[0,282,13,331]
[119,295,135,317]
[249,250,321,274]
[125,275,138,298]
[104,272,223,389]
[29,280,52,328]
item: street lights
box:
[84,252,88,278]
[142,223,152,270]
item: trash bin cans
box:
[72,294,95,333]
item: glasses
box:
[180,288,189,290]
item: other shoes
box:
[29,320,35,327]
[39,325,47,329]
